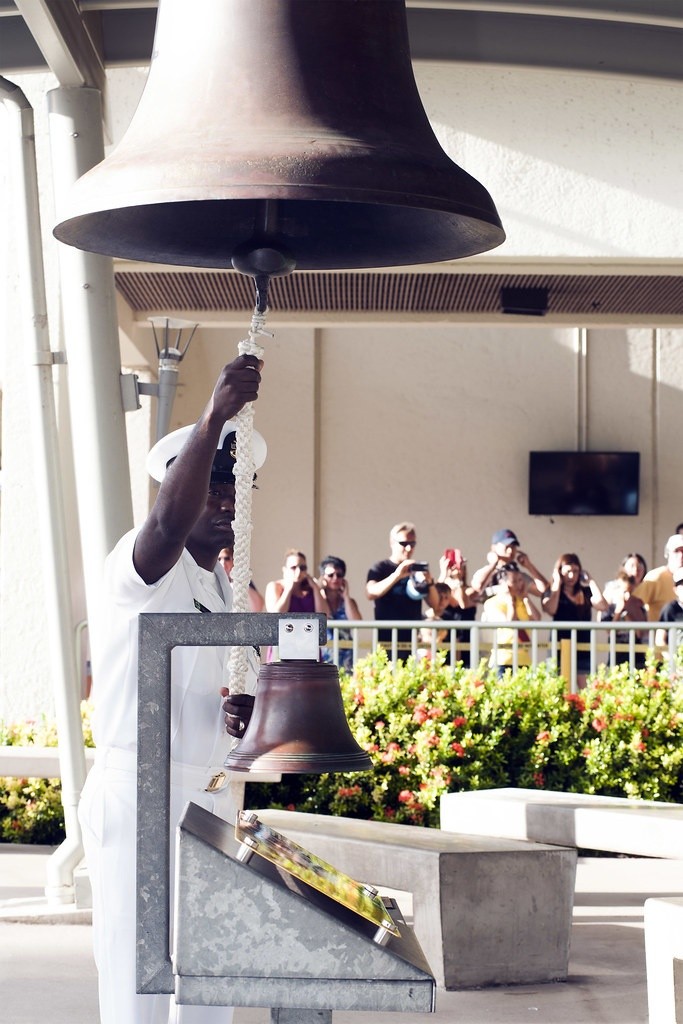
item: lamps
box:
[134,315,200,488]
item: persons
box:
[215,546,265,612]
[265,549,331,663]
[422,528,549,682]
[365,522,440,668]
[539,553,608,688]
[317,555,362,672]
[77,350,263,1024]
[596,521,683,672]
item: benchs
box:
[440,788,683,857]
[252,806,576,990]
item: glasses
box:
[323,573,344,578]
[218,556,231,562]
[395,541,416,548]
[286,564,307,571]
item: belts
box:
[94,746,231,793]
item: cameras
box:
[409,563,428,571]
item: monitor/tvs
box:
[527,451,640,515]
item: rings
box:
[238,721,245,730]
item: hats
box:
[148,422,267,490]
[672,567,683,582]
[668,534,683,552]
[492,529,520,547]
